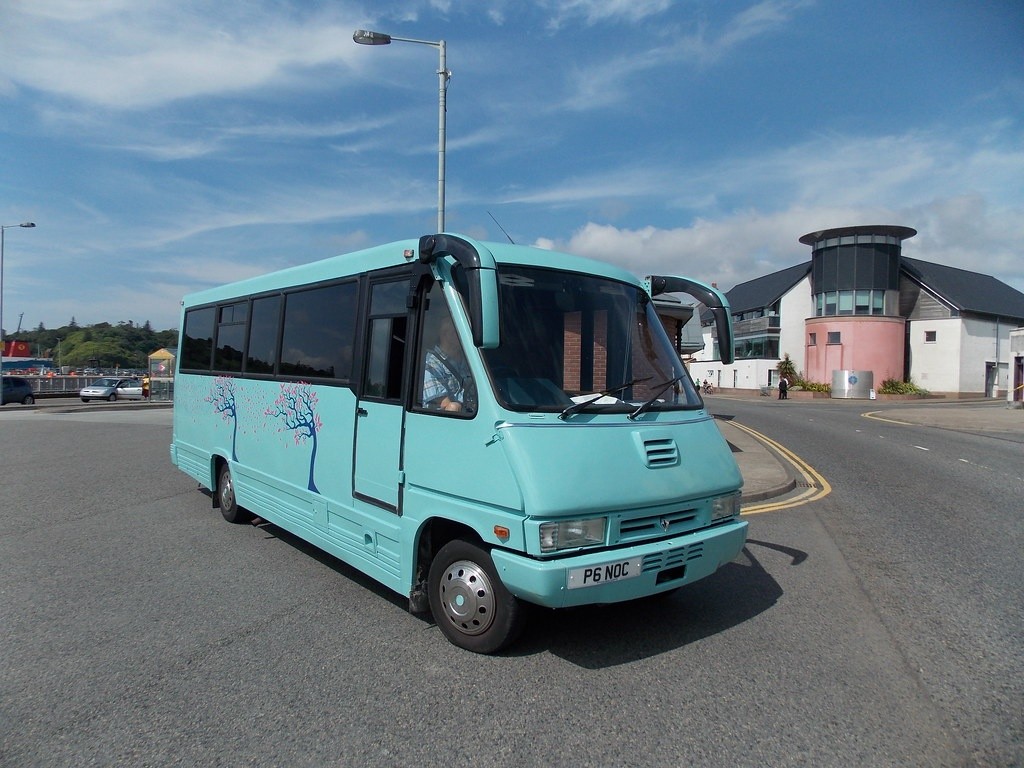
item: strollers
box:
[703,385,713,395]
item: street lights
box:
[0,222,36,330]
[354,30,447,234]
[57,338,61,374]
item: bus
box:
[169,233,750,654]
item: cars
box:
[76,368,148,376]
[80,378,142,402]
[1,375,36,405]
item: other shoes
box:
[778,397,787,399]
[704,393,706,394]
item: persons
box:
[703,379,713,395]
[695,378,700,392]
[420,315,508,414]
[141,373,150,399]
[128,374,139,401]
[778,375,788,400]
